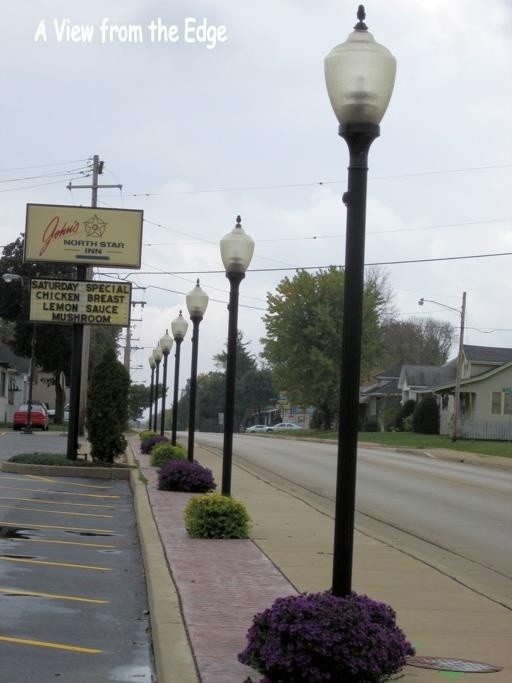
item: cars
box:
[13,398,70,431]
[261,423,303,432]
[245,425,268,433]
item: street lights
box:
[171,309,188,447]
[148,353,157,430]
[185,277,208,463]
[418,291,467,442]
[153,341,163,432]
[220,215,254,496]
[159,330,174,437]
[322,4,396,598]
